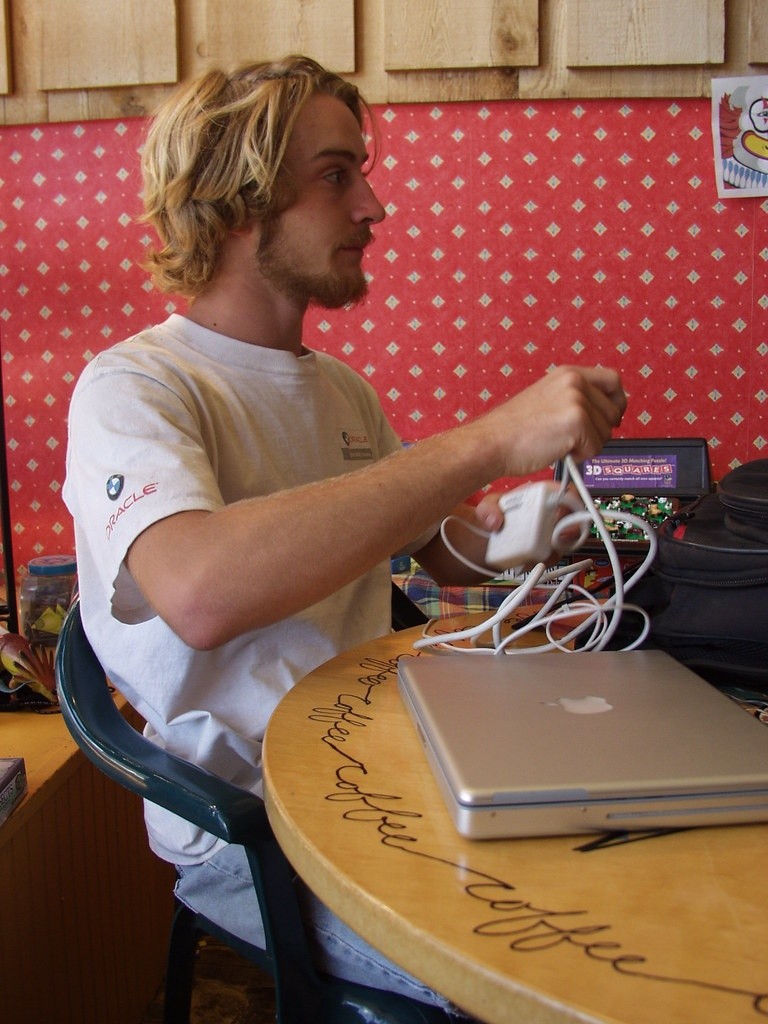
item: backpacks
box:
[573,459,768,706]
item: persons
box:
[59,56,627,1016]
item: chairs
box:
[52,575,458,1024]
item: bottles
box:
[19,553,77,647]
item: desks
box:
[260,598,768,1024]
[0,677,177,1024]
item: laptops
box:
[396,649,768,838]
